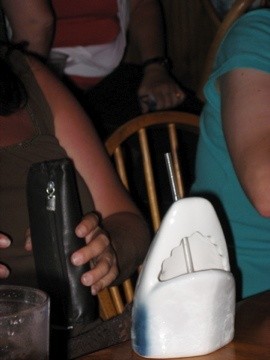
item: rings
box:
[96,257,112,268]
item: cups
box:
[48,51,69,80]
[0,285,48,360]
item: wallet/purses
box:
[27,158,99,328]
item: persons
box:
[0,0,186,296]
[187,7,270,299]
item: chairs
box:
[82,111,201,320]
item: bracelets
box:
[141,56,174,70]
